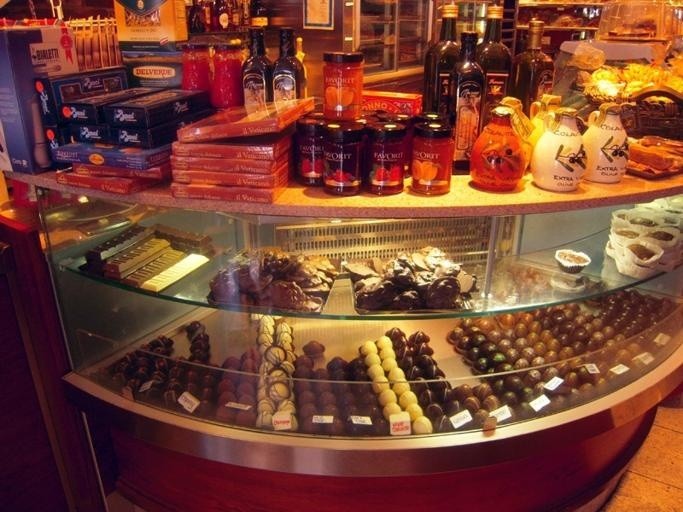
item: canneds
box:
[181,42,244,108]
[293,51,455,196]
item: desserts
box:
[108,267,680,437]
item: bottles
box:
[270,28,307,104]
[475,5,514,133]
[422,4,462,124]
[240,26,273,103]
[442,31,484,173]
[508,21,554,118]
[30,102,51,169]
[470,93,626,192]
[187,0,269,33]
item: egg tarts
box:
[606,194,683,267]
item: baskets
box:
[584,70,683,137]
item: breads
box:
[209,245,336,314]
[338,244,480,309]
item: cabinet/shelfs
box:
[343,1,435,84]
[22,169,681,511]
[498,1,518,60]
